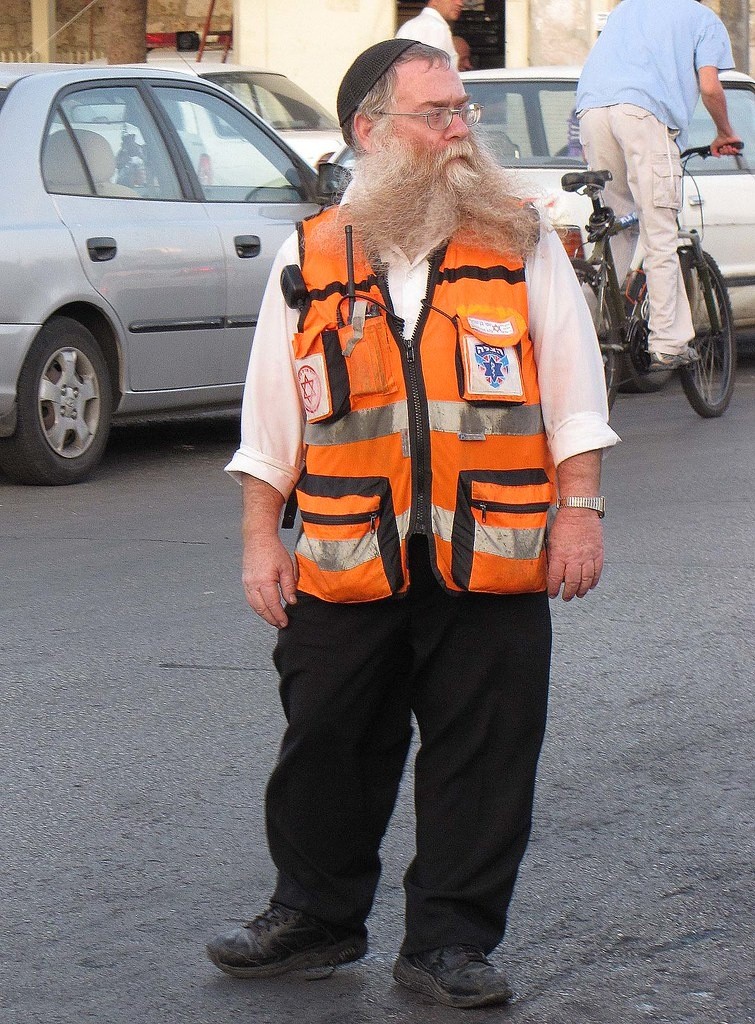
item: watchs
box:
[556,495,605,519]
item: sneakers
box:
[648,348,705,372]
[205,899,368,982]
[392,944,512,1008]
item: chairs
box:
[42,129,141,198]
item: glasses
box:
[386,103,484,132]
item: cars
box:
[457,67,755,393]
[0,64,353,486]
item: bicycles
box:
[561,142,745,418]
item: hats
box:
[338,39,417,128]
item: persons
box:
[395,0,464,73]
[223,39,623,1008]
[576,0,743,373]
[452,36,473,72]
